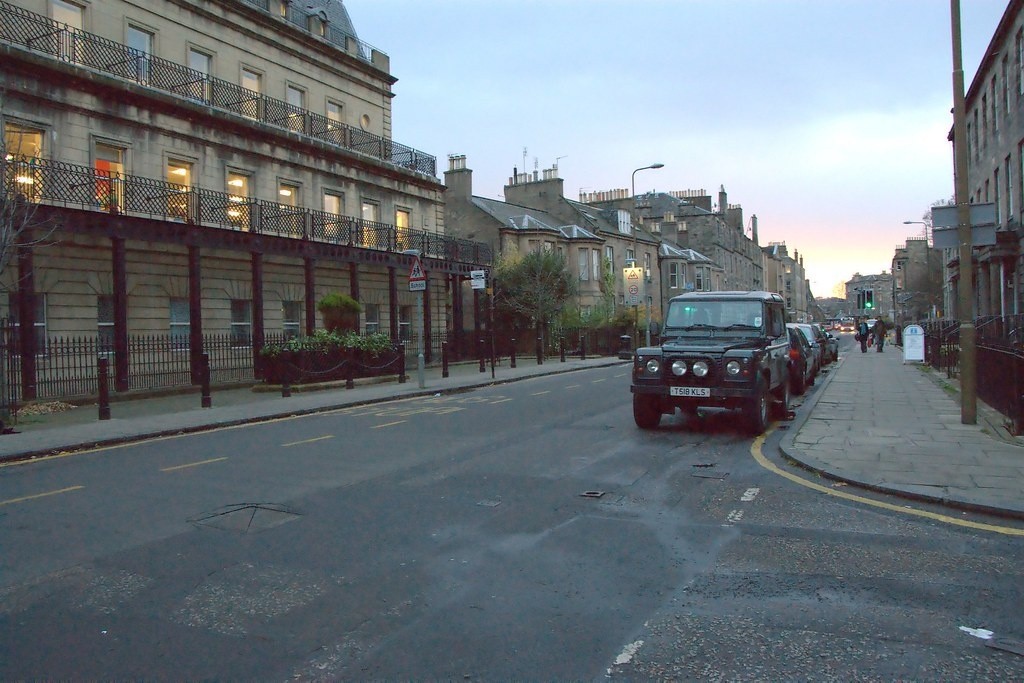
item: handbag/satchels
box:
[855,333,860,341]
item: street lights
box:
[632,162,666,355]
[903,219,933,345]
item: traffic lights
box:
[864,288,874,309]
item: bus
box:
[838,316,856,331]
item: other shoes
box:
[877,348,882,352]
[862,348,867,352]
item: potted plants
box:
[262,333,397,386]
[316,292,363,335]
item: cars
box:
[866,319,878,330]
[787,320,840,395]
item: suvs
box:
[628,289,800,434]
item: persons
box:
[856,319,869,352]
[874,316,887,352]
